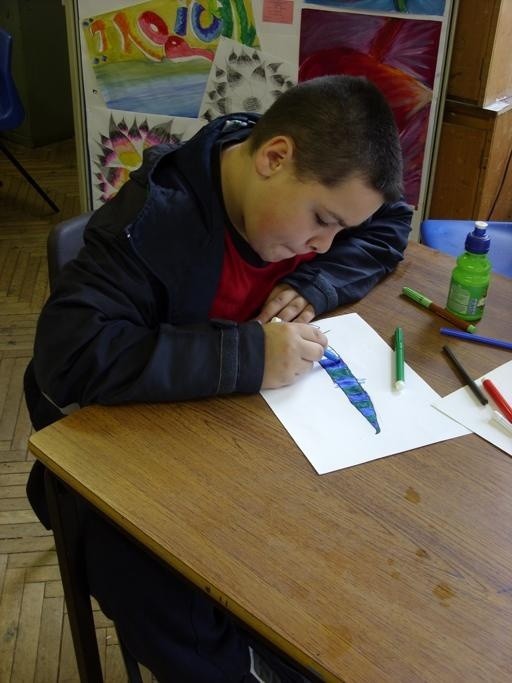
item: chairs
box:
[1,28,60,213]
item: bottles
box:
[446,220,492,323]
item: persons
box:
[17,69,416,681]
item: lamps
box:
[25,242,512,683]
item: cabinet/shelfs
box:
[446,0,512,108]
[429,99,512,222]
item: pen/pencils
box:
[270,316,340,363]
[439,327,512,350]
[442,345,488,406]
[492,411,512,432]
[395,327,405,390]
[402,286,476,334]
[481,376,512,423]
